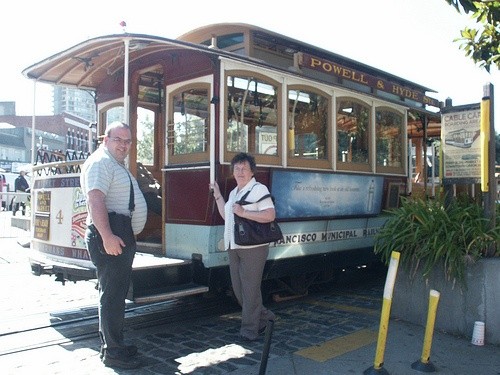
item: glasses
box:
[232,167,252,172]
[109,135,133,145]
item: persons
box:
[80,122,147,370]
[209,152,276,344]
[12,171,30,216]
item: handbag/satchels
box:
[233,185,284,245]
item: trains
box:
[19,23,489,312]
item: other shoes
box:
[99,345,138,361]
[237,336,254,343]
[22,211,25,216]
[258,325,268,335]
[13,211,16,215]
[102,350,142,369]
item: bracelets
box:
[215,195,221,200]
